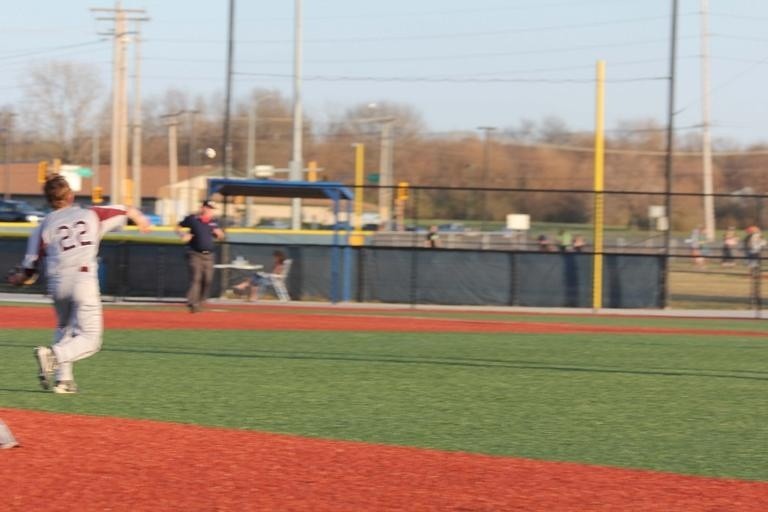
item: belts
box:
[197,250,210,254]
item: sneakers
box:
[33,346,54,389]
[51,382,77,395]
[190,305,201,313]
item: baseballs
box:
[203,147,216,160]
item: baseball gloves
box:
[6,260,41,286]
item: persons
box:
[22,176,152,397]
[174,199,226,311]
[536,234,553,251]
[555,228,572,246]
[426,224,440,240]
[742,225,766,266]
[572,233,587,250]
[233,249,287,303]
[685,223,707,265]
[720,224,739,266]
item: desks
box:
[212,261,260,304]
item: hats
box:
[204,200,220,209]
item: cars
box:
[0,199,46,221]
[404,223,466,234]
[326,221,351,229]
[255,217,310,230]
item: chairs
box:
[253,256,293,303]
